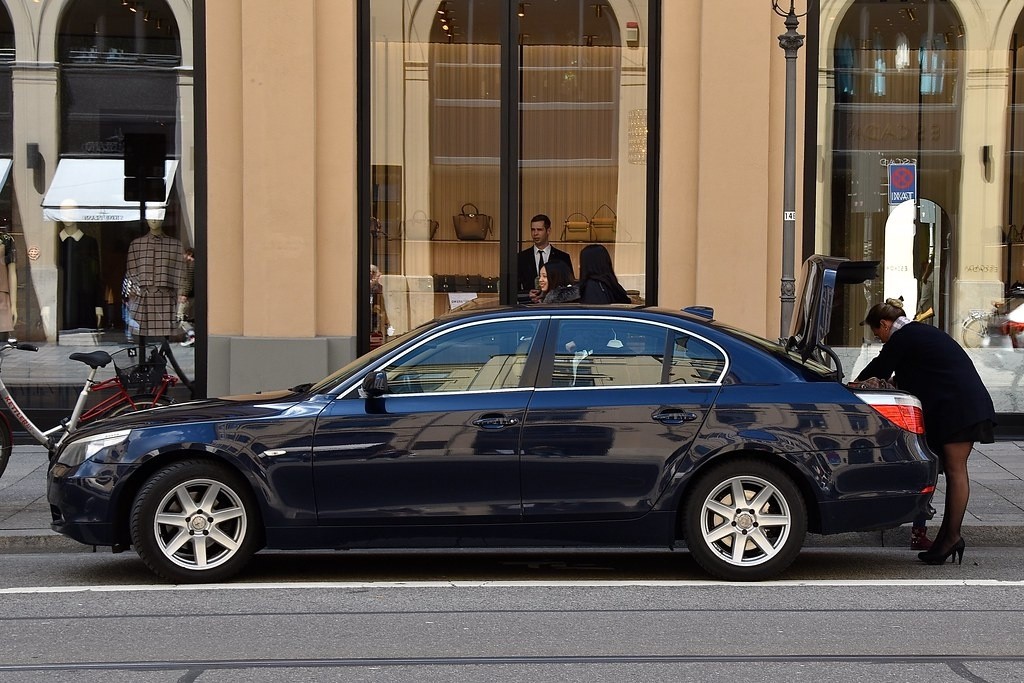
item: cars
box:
[47,256,940,585]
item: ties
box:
[538,250,544,276]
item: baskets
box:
[111,345,167,388]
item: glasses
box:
[874,324,882,340]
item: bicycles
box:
[0,337,179,480]
[962,289,1024,348]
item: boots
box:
[911,526,934,550]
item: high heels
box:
[918,538,965,565]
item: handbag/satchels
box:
[453,203,494,240]
[1006,226,1023,243]
[590,204,617,242]
[399,210,439,240]
[455,274,481,291]
[481,277,499,293]
[433,274,456,292]
[560,213,591,242]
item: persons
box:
[176,247,195,346]
[518,214,574,302]
[371,265,394,337]
[125,201,187,342]
[566,244,631,352]
[0,232,18,341]
[533,260,581,303]
[853,294,995,565]
[55,199,105,332]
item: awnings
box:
[40,158,179,221]
